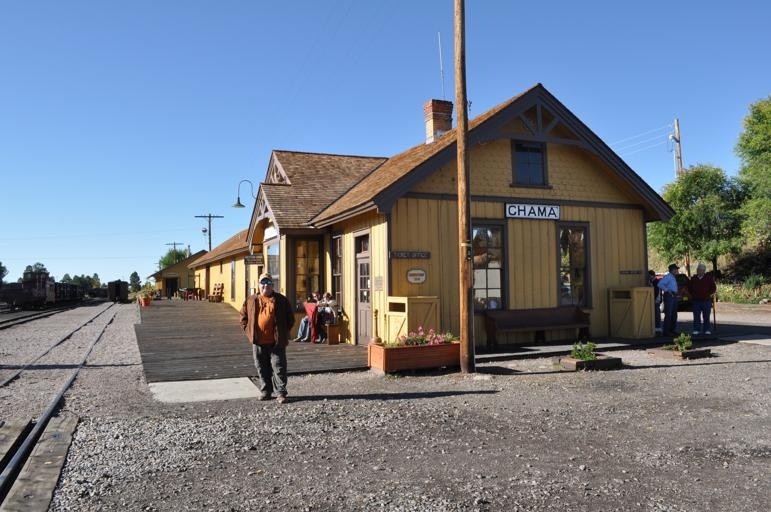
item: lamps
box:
[231,178,264,209]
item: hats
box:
[668,263,680,270]
[258,273,272,281]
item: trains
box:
[0,272,128,310]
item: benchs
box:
[322,323,340,345]
[207,283,224,303]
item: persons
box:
[238,272,296,404]
[687,263,717,336]
[292,291,325,342]
[313,292,338,343]
[648,269,662,333]
[657,263,680,336]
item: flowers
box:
[397,325,457,348]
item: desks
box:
[183,288,201,301]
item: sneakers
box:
[293,336,327,343]
[663,331,680,337]
[258,394,289,403]
[692,331,711,335]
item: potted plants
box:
[136,291,151,306]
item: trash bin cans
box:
[385,296,441,345]
[608,287,656,340]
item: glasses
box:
[261,280,272,285]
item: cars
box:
[653,273,692,311]
